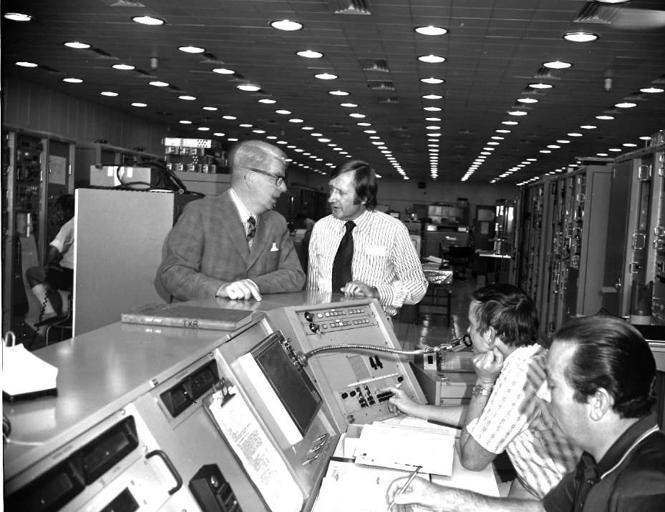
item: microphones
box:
[440,334,472,353]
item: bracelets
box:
[471,384,493,399]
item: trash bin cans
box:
[476,272,498,290]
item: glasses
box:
[254,170,285,186]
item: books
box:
[310,416,464,510]
[120,302,252,331]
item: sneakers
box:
[33,311,58,327]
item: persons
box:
[25,191,75,328]
[152,136,307,302]
[378,281,582,501]
[304,160,430,319]
[383,313,665,511]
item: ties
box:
[247,217,256,251]
[332,220,356,292]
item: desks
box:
[414,231,512,327]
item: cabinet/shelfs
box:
[1,125,330,345]
[494,142,665,346]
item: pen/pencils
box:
[386,465,423,511]
